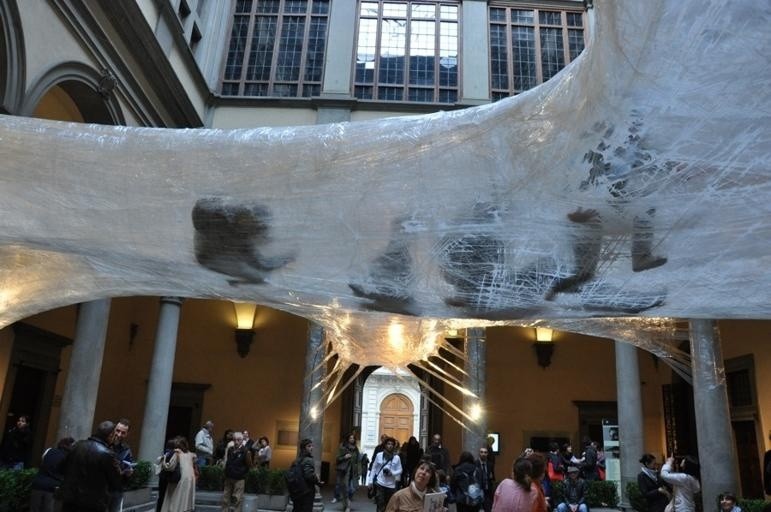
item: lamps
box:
[488,431,500,456]
[233,300,257,358]
[532,326,555,368]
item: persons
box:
[1,414,771,511]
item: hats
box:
[567,466,580,473]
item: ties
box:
[482,464,486,472]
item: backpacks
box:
[461,471,485,507]
[284,454,314,502]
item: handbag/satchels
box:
[159,456,181,483]
[368,477,378,499]
[597,466,605,480]
[663,495,675,512]
[334,453,351,473]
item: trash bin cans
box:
[320,460,330,486]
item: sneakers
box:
[330,494,341,504]
[344,498,352,512]
